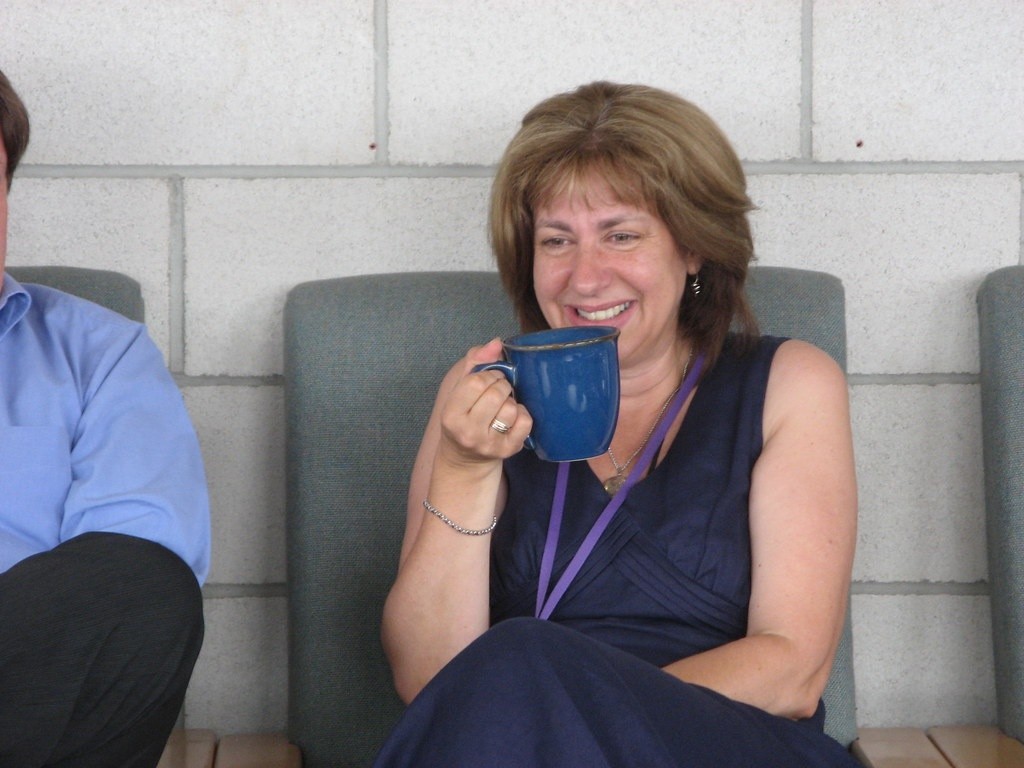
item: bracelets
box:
[421,499,498,536]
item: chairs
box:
[284,265,858,768]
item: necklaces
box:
[597,334,696,495]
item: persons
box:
[3,71,215,767]
[376,79,867,768]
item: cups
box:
[466,325,620,462]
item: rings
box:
[490,419,512,433]
